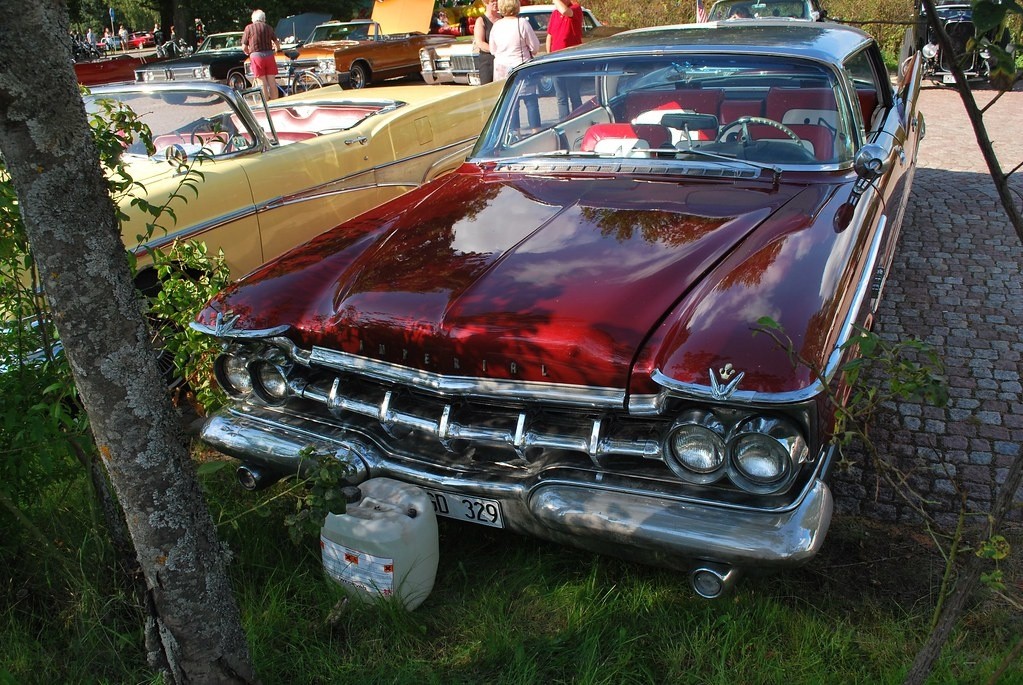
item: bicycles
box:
[243,49,323,95]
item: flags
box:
[697,0,708,23]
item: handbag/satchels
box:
[524,76,538,87]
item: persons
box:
[545,0,585,124]
[284,35,299,44]
[434,11,449,26]
[242,9,281,101]
[474,0,502,87]
[459,14,466,36]
[488,0,542,133]
[67,17,241,62]
[727,7,757,20]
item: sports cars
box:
[0,77,507,377]
[243,20,458,96]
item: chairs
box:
[581,123,671,157]
[230,132,319,152]
[155,132,229,155]
[738,125,835,162]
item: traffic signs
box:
[109,8,114,18]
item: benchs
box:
[622,87,880,159]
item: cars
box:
[418,3,604,86]
[914,4,1017,89]
[705,0,820,23]
[91,29,156,51]
[188,17,926,599]
[133,30,301,94]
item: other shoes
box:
[123,52,126,53]
[127,51,130,54]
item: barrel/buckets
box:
[320,478,436,611]
